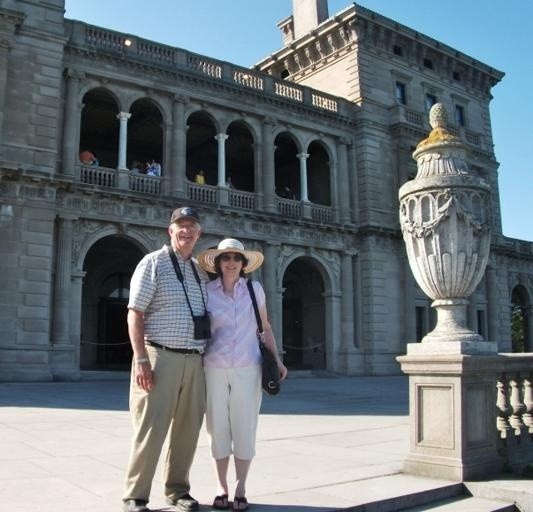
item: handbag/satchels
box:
[258,335,281,397]
[192,313,212,340]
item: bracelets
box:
[133,354,150,363]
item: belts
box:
[146,339,205,357]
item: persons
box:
[282,185,295,200]
[225,175,235,190]
[194,169,206,185]
[80,145,162,177]
[120,207,213,512]
[195,237,288,511]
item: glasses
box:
[221,255,242,261]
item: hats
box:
[196,238,265,274]
[170,206,202,224]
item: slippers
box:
[232,497,249,512]
[213,494,229,510]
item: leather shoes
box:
[122,499,149,512]
[167,493,199,512]
